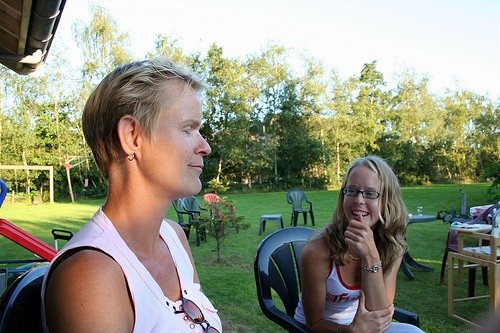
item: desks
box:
[402,215,436,281]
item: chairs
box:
[172,193,238,245]
[287,190,315,227]
[439,204,500,328]
[254,227,419,333]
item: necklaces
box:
[347,250,360,260]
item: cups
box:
[417,206,423,216]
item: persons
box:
[42,58,223,333]
[294,156,426,333]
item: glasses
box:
[341,187,380,200]
[174,297,220,333]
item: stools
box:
[258,214,284,236]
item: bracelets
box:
[361,260,382,273]
